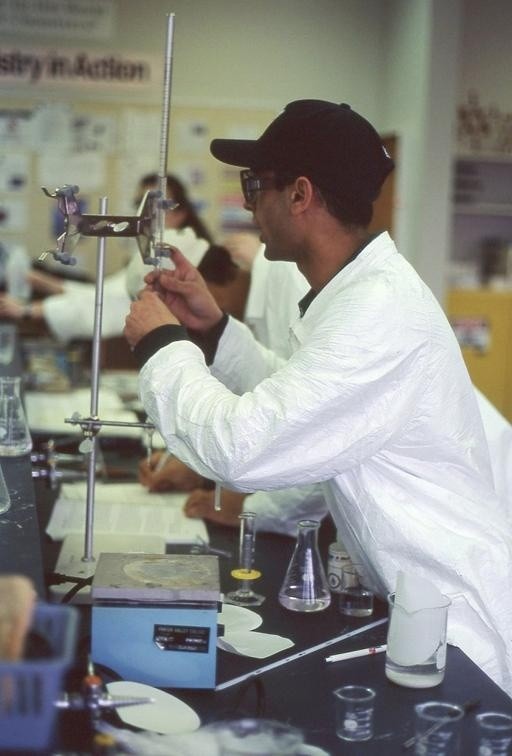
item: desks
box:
[2,354,512,756]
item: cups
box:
[383,591,452,688]
[324,540,375,618]
[333,685,375,743]
[415,700,464,756]
[474,712,511,756]
[214,716,301,756]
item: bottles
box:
[227,511,265,606]
[1,377,34,458]
[279,520,333,614]
[0,467,12,515]
[153,245,173,306]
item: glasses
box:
[237,170,288,205]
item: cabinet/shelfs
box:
[447,280,512,426]
[453,141,510,215]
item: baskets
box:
[0,602,78,752]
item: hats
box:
[208,98,396,205]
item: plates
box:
[215,604,264,634]
[102,680,203,736]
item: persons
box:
[122,96,512,699]
[130,226,329,537]
[0,172,210,342]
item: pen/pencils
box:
[139,450,173,496]
[322,644,386,663]
